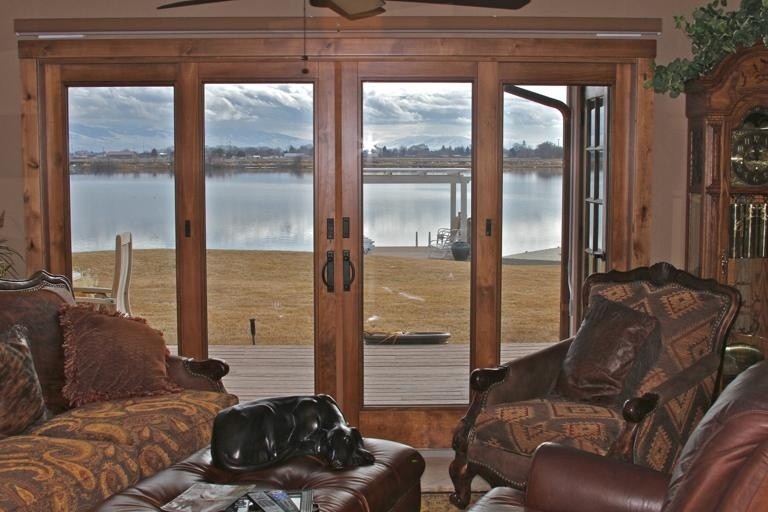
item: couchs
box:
[0,273,240,512]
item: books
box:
[157,481,257,510]
[729,201,767,257]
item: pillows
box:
[558,295,663,411]
[0,305,167,436]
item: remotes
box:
[247,492,284,512]
[264,490,299,512]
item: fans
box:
[157,0,529,18]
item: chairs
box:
[464,359,768,511]
[451,263,744,508]
[427,228,462,260]
[74,232,133,317]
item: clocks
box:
[684,45,768,346]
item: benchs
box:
[93,431,425,512]
